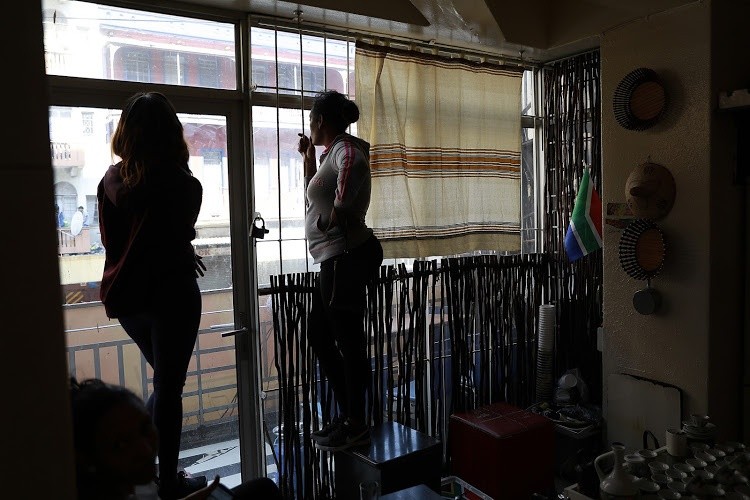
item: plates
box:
[683,423,717,440]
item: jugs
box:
[595,445,641,500]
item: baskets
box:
[618,218,671,282]
[612,67,670,133]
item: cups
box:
[648,462,669,475]
[705,449,727,459]
[665,468,687,481]
[639,481,660,495]
[673,463,695,475]
[650,474,673,489]
[359,480,379,500]
[558,375,578,388]
[715,444,734,455]
[694,452,716,465]
[668,481,683,491]
[690,414,711,426]
[693,461,726,480]
[728,441,745,452]
[657,488,682,500]
[665,428,689,456]
[624,455,644,477]
[724,456,733,462]
[685,458,707,470]
[638,449,658,471]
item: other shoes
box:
[311,417,370,451]
[157,475,208,500]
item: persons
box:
[296,90,385,453]
[78,206,89,227]
[69,377,158,500]
[98,93,209,500]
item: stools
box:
[449,403,556,500]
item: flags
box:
[566,168,603,260]
